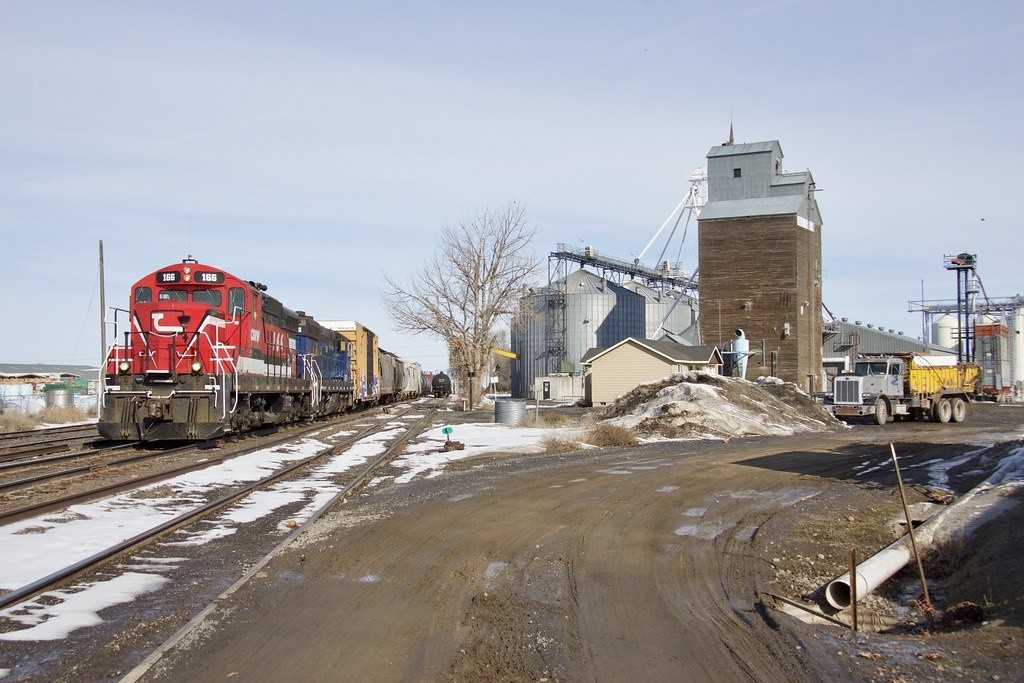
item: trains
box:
[431,370,452,398]
[95,255,433,443]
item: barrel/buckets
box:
[495,402,525,427]
[46,390,73,409]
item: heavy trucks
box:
[821,351,983,425]
